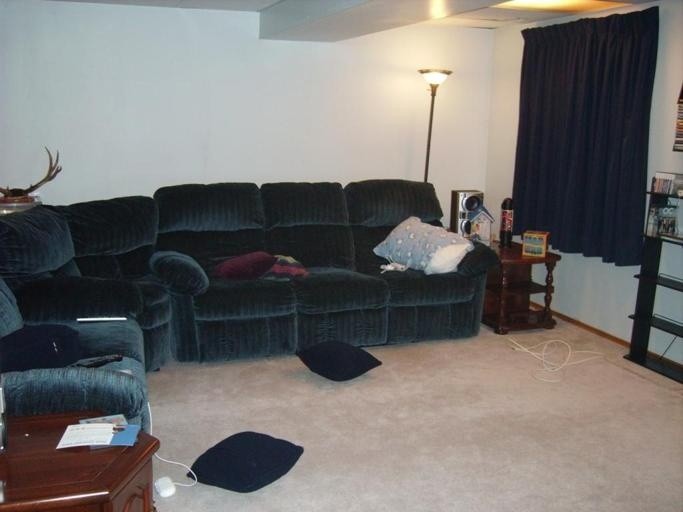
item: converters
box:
[154,476,177,498]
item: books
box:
[55,414,142,448]
[646,205,677,238]
[76,302,130,321]
[655,171,683,195]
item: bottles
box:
[499,196,514,248]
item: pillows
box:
[0,323,79,370]
[373,216,475,274]
[216,249,275,278]
[296,342,381,381]
[150,252,209,296]
[187,430,304,495]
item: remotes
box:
[77,354,122,367]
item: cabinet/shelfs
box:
[623,174,683,386]
[0,413,159,512]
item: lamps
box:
[416,67,453,182]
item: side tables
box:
[481,239,561,334]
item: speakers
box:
[451,190,483,238]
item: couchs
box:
[0,181,500,423]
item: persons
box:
[672,85,683,152]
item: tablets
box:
[76,313,127,321]
